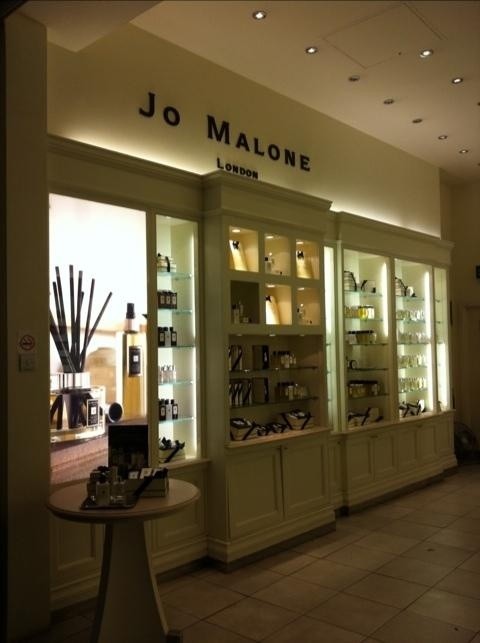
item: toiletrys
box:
[157,290,179,421]
[344,303,426,397]
[228,299,316,407]
[86,465,131,507]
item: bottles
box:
[274,380,306,401]
[156,287,180,310]
[345,328,376,345]
[233,300,251,322]
[263,256,282,274]
[157,364,178,387]
[397,308,429,394]
[272,350,297,368]
[157,325,179,349]
[114,301,144,423]
[297,304,312,325]
[158,395,179,421]
[345,379,381,398]
[343,302,375,321]
[95,472,124,505]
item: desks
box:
[47,475,203,642]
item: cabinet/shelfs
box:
[337,212,394,507]
[393,225,456,491]
[156,214,201,466]
[203,171,333,562]
